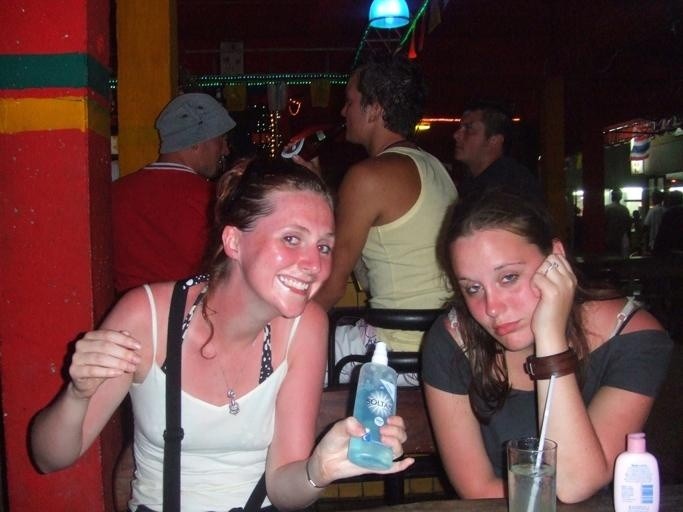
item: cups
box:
[508,437,556,512]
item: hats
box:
[155,93,237,153]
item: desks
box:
[343,474,683,512]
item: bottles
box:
[276,123,345,163]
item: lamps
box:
[368,1,410,30]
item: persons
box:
[593,207,630,257]
[418,187,674,504]
[642,190,667,252]
[29,157,415,512]
[111,92,237,297]
[452,102,545,211]
[311,57,459,387]
[604,189,632,233]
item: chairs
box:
[318,307,443,503]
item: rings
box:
[544,262,559,276]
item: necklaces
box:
[209,337,257,416]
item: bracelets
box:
[522,347,580,381]
[305,459,329,489]
